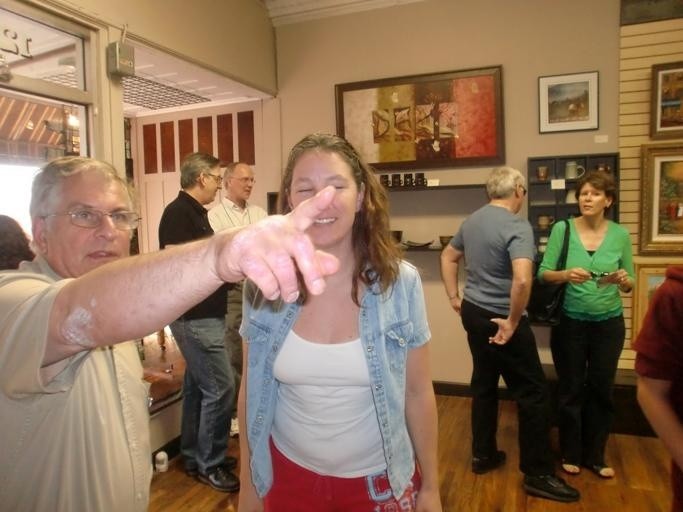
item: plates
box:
[527,152,620,263]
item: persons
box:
[209,161,270,437]
[442,168,582,500]
[636,260,682,512]
[236,133,439,512]
[538,172,635,478]
[0,216,35,269]
[160,154,241,492]
[1,158,338,511]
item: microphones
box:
[533,365,661,437]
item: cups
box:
[517,184,529,197]
[37,209,143,232]
[227,175,257,185]
[588,271,611,281]
[203,173,224,185]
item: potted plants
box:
[634,261,683,348]
[537,71,598,133]
[650,61,683,141]
[336,66,508,169]
[640,142,683,254]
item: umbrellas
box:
[583,458,617,479]
[561,455,581,474]
[189,462,242,495]
[183,455,239,477]
[469,450,506,475]
[522,470,581,503]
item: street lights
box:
[527,220,570,328]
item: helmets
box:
[401,239,435,248]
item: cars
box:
[391,174,402,186]
[565,161,585,179]
[537,215,555,229]
[379,174,390,187]
[439,236,453,247]
[390,231,402,243]
[414,173,427,186]
[403,173,415,186]
[536,166,548,182]
